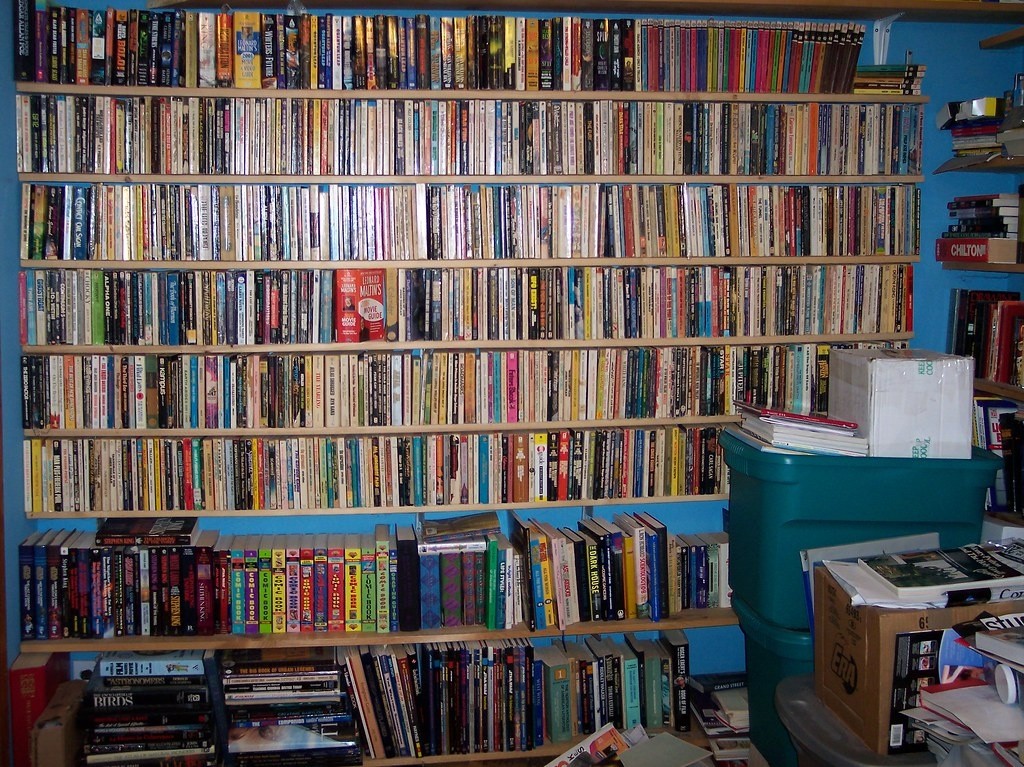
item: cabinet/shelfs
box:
[16,1,1024,767]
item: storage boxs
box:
[716,349,1024,767]
[31,679,86,767]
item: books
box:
[10,1,1023,767]
